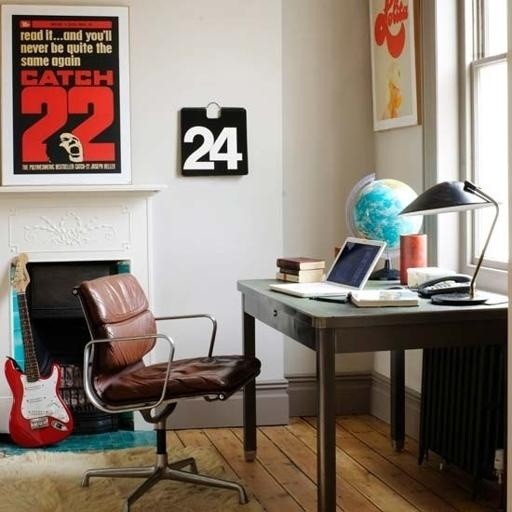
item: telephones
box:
[418,274,475,297]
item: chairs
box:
[71,271,263,512]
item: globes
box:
[345,173,424,280]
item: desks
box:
[236,274,511,512]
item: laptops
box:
[270,237,386,297]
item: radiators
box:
[415,340,507,500]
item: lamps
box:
[397,178,511,307]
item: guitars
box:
[5,253,73,449]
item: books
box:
[350,289,421,307]
[276,257,326,283]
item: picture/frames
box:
[366,0,422,132]
[0,2,135,190]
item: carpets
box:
[0,444,268,512]
